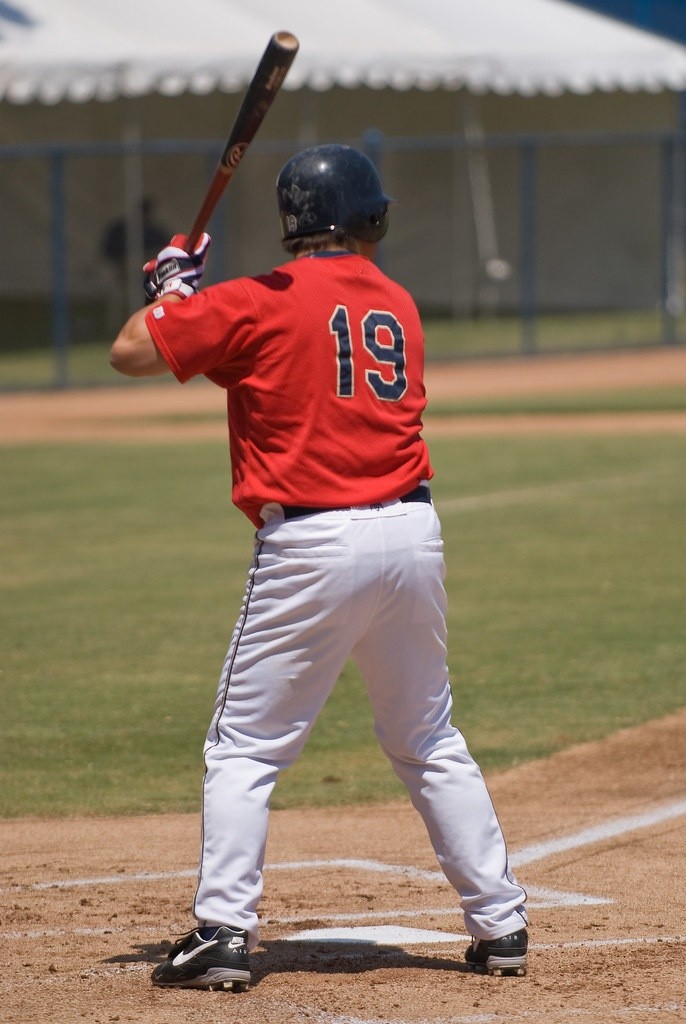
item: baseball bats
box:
[184,29,300,262]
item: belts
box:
[280,486,432,520]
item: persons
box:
[105,142,530,988]
[98,196,170,288]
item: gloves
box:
[142,258,160,306]
[154,231,211,300]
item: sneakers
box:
[463,927,529,976]
[149,926,253,991]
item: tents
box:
[0,0,686,385]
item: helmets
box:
[275,142,393,241]
